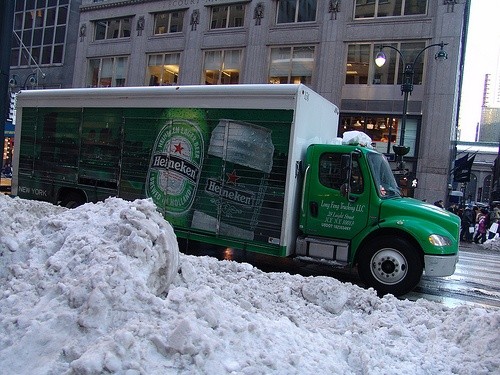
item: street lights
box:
[9,72,36,91]
[375,41,450,187]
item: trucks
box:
[12,83,461,297]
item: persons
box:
[423,199,500,245]
[0,162,13,190]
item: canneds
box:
[190,118,275,242]
[144,108,209,228]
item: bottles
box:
[139,108,210,231]
